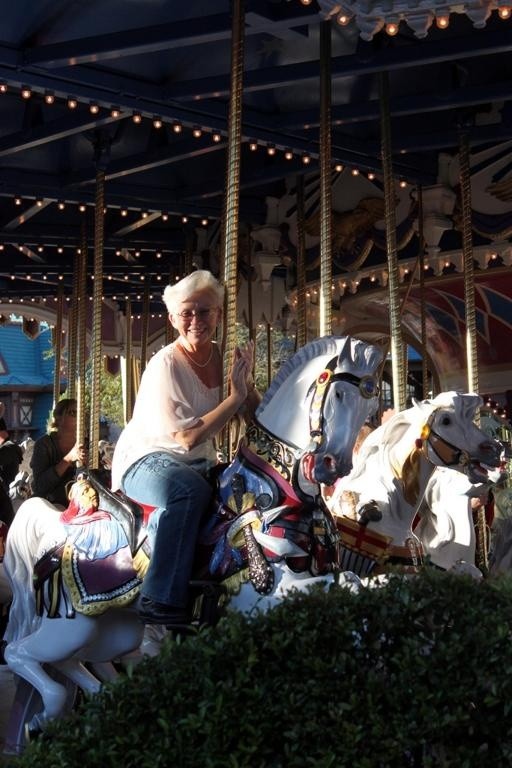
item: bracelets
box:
[249,382,258,394]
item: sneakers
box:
[137,598,188,626]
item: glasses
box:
[173,307,219,318]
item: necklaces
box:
[181,342,214,368]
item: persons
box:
[105,268,264,620]
[1,417,24,528]
[29,399,88,508]
[60,479,143,616]
